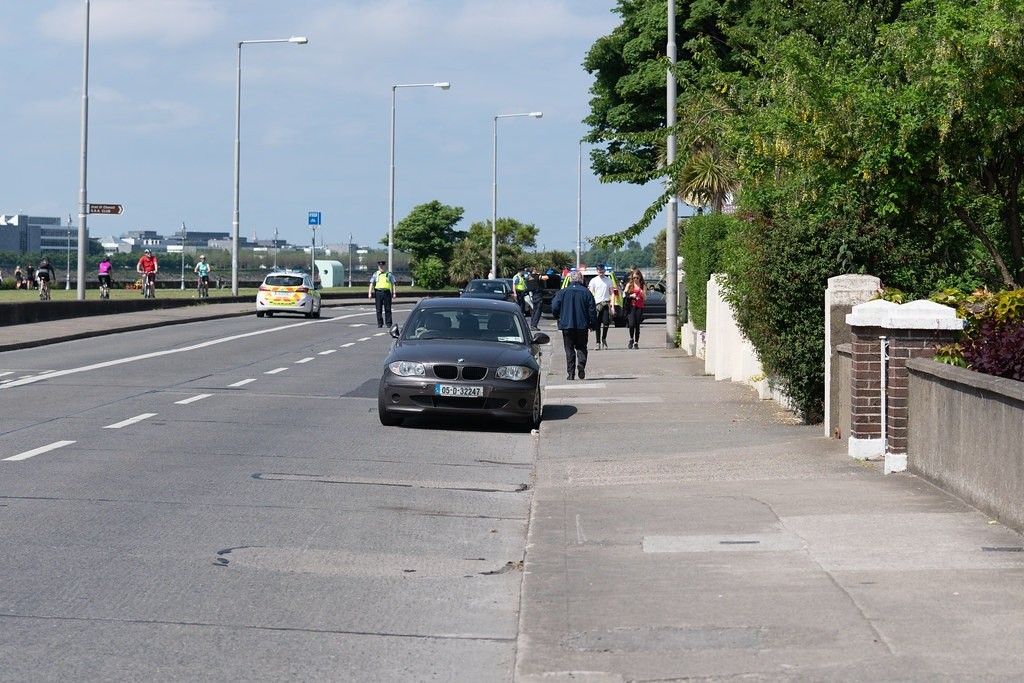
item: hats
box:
[377,260,386,265]
[516,267,525,271]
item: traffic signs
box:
[88,203,124,215]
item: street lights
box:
[348,232,353,287]
[181,222,187,290]
[66,214,73,290]
[490,111,543,281]
[388,82,451,273]
[274,227,279,271]
[230,36,308,298]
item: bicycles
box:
[100,278,114,299]
[14,275,37,290]
[139,271,158,299]
[39,276,56,301]
[195,270,207,298]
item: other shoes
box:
[387,325,391,328]
[602,342,608,350]
[567,373,574,380]
[595,342,601,350]
[628,339,634,349]
[378,324,382,327]
[634,343,639,349]
[577,364,585,379]
[530,326,540,331]
[141,290,145,294]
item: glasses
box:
[633,277,640,279]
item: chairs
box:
[421,310,513,340]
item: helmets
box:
[144,248,151,252]
[200,254,206,258]
[41,255,50,264]
[103,256,110,261]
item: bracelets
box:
[393,293,396,294]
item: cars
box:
[256,271,323,319]
[459,279,514,320]
[378,298,551,432]
[495,265,667,328]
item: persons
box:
[552,271,598,380]
[97,255,112,299]
[547,268,554,274]
[194,255,210,297]
[563,266,569,277]
[15,266,23,289]
[524,268,530,277]
[650,285,654,294]
[527,267,549,331]
[589,264,615,350]
[137,248,158,298]
[25,263,35,290]
[624,269,647,349]
[35,266,40,284]
[620,265,637,292]
[513,267,526,307]
[368,261,396,328]
[39,256,56,300]
